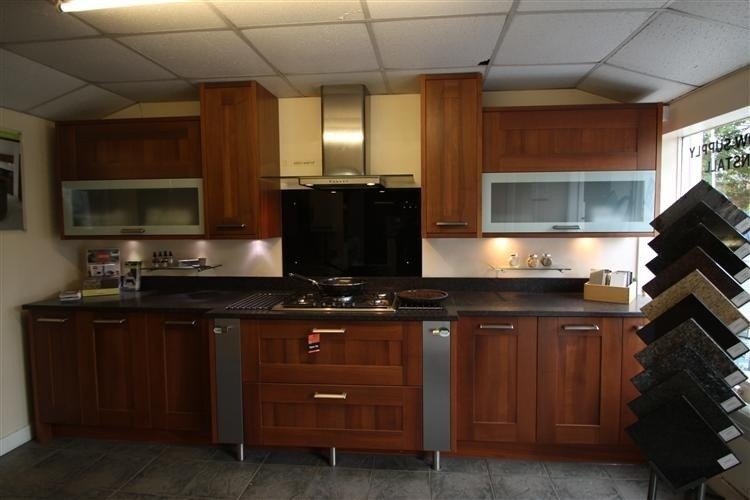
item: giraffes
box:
[278,289,399,313]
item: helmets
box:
[398,288,449,303]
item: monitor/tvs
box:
[58,248,140,301]
[589,266,633,288]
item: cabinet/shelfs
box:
[527,253,539,267]
[152,250,174,267]
[509,253,520,266]
[542,252,553,266]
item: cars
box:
[198,257,207,266]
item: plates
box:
[28,309,210,449]
[480,173,662,239]
[456,316,654,452]
[57,178,204,240]
[418,70,483,239]
[242,383,424,452]
[56,115,201,179]
[197,79,283,241]
[240,318,424,386]
[480,104,664,173]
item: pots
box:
[289,272,368,295]
